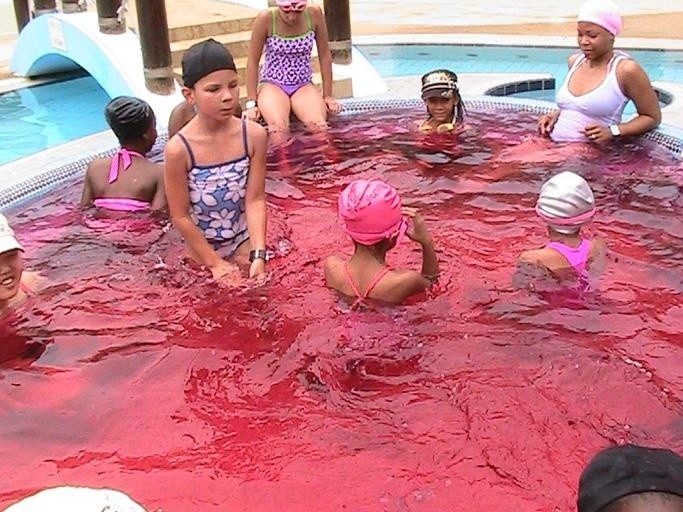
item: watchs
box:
[244,98,257,109]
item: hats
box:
[577,1,622,36]
[182,38,237,88]
[338,177,402,246]
[0,214,26,254]
[536,171,596,235]
[421,70,459,101]
[104,96,154,141]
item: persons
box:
[169,101,241,137]
[240,0,341,170]
[81,96,168,211]
[518,172,608,283]
[324,180,439,304]
[0,214,53,317]
[538,8,662,145]
[161,38,268,285]
[414,70,471,136]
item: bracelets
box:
[609,123,623,140]
[249,250,268,260]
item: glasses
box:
[276,0,307,12]
[418,117,457,133]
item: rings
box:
[592,134,596,139]
